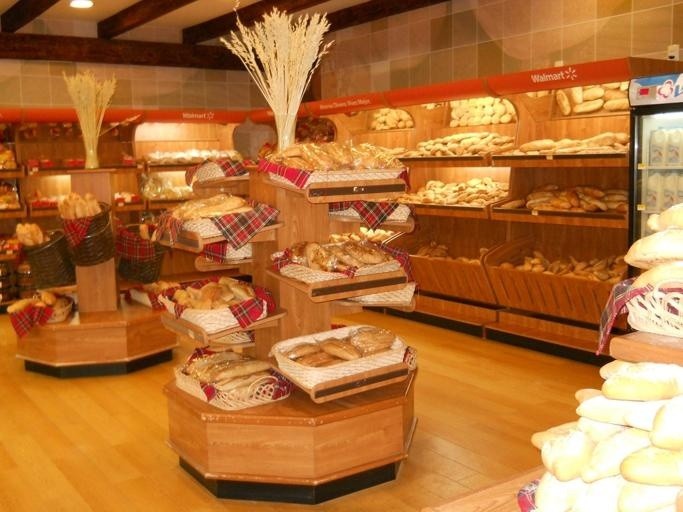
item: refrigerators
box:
[626,72,683,291]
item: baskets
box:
[128,288,152,308]
[624,279,683,338]
[46,295,74,324]
[271,324,408,390]
[157,292,267,334]
[181,217,224,240]
[21,230,78,289]
[347,281,415,306]
[118,223,168,283]
[328,201,411,223]
[56,201,115,266]
[194,161,225,181]
[268,165,406,190]
[213,333,255,345]
[269,241,402,287]
[172,350,291,411]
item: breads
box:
[0,183,23,211]
[518,132,631,156]
[503,184,630,215]
[6,290,75,318]
[619,199,683,329]
[527,90,551,97]
[151,228,159,241]
[56,192,102,218]
[449,98,518,127]
[522,354,683,512]
[171,276,255,311]
[288,237,383,272]
[142,279,179,292]
[500,250,633,284]
[187,351,275,391]
[16,222,43,247]
[369,108,413,131]
[406,241,487,264]
[264,134,404,175]
[137,222,150,240]
[280,326,397,370]
[0,149,20,172]
[144,147,241,164]
[172,194,252,218]
[143,183,194,199]
[555,83,630,115]
[401,177,507,207]
[404,131,515,157]
